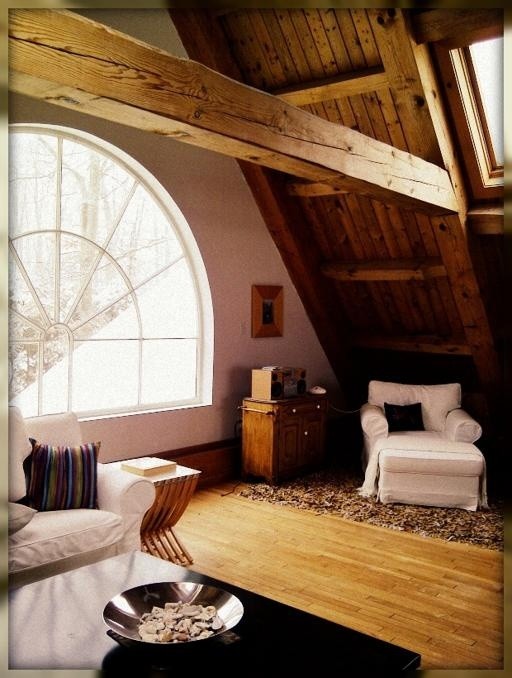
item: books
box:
[120,456,177,477]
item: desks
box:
[9,550,421,678]
[106,456,202,568]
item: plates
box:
[102,581,245,646]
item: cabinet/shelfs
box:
[242,396,327,482]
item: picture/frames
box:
[250,285,283,338]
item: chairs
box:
[359,380,490,512]
[9,409,156,584]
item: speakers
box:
[252,369,283,400]
[295,367,308,396]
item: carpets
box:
[240,474,505,559]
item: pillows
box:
[9,502,35,537]
[23,437,101,510]
[384,402,426,432]
